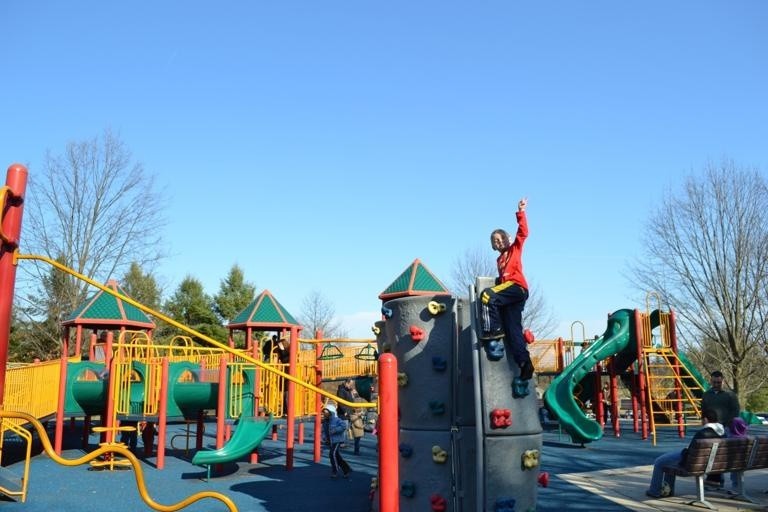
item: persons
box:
[272,338,290,417]
[118,374,142,454]
[322,404,354,480]
[699,371,743,496]
[666,390,677,422]
[141,421,159,456]
[99,331,114,359]
[32,356,41,364]
[262,334,278,362]
[645,406,727,498]
[702,416,748,487]
[478,195,536,386]
[321,376,381,456]
[601,380,614,425]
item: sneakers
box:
[330,467,351,480]
[705,485,744,495]
[519,360,535,380]
[646,490,674,498]
[477,327,505,340]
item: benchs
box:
[661,435,768,510]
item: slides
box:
[191,411,273,465]
[543,308,633,442]
[657,343,762,424]
